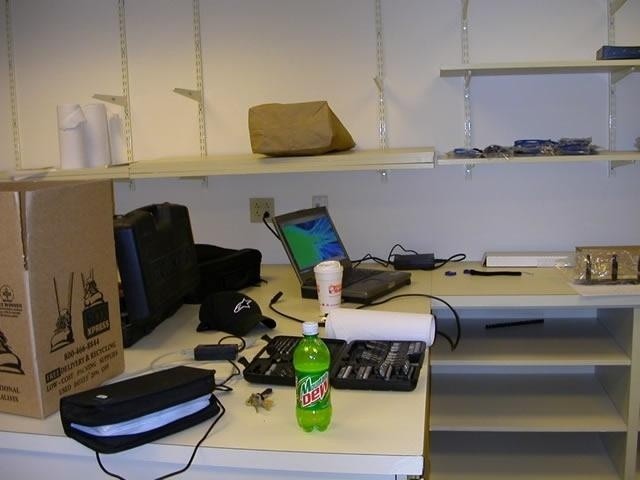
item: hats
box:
[197,290,277,337]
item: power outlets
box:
[250,198,275,223]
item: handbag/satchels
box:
[248,100,356,156]
[60,365,220,454]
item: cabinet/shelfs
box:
[432,307,640,475]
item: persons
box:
[49,267,104,353]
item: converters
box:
[195,344,237,362]
[393,253,435,270]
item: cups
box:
[314,259,345,314]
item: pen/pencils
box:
[108,163,129,167]
[485,318,544,329]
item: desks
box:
[2,262,639,478]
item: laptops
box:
[272,205,411,303]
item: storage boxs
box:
[0,180,126,420]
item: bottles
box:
[293,320,331,433]
